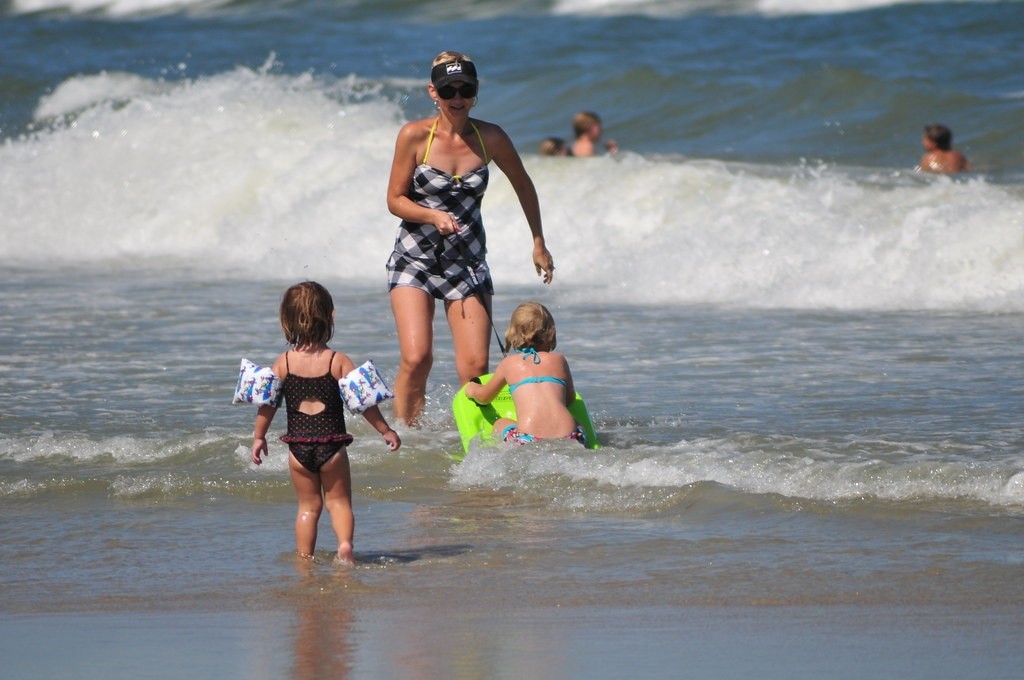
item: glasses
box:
[437,83,475,100]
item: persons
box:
[541,138,568,156]
[251,281,401,554]
[465,302,587,446]
[919,124,969,173]
[386,52,552,426]
[573,112,618,157]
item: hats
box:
[431,61,477,89]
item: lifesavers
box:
[233,357,282,407]
[337,358,396,415]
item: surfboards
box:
[451,374,600,458]
